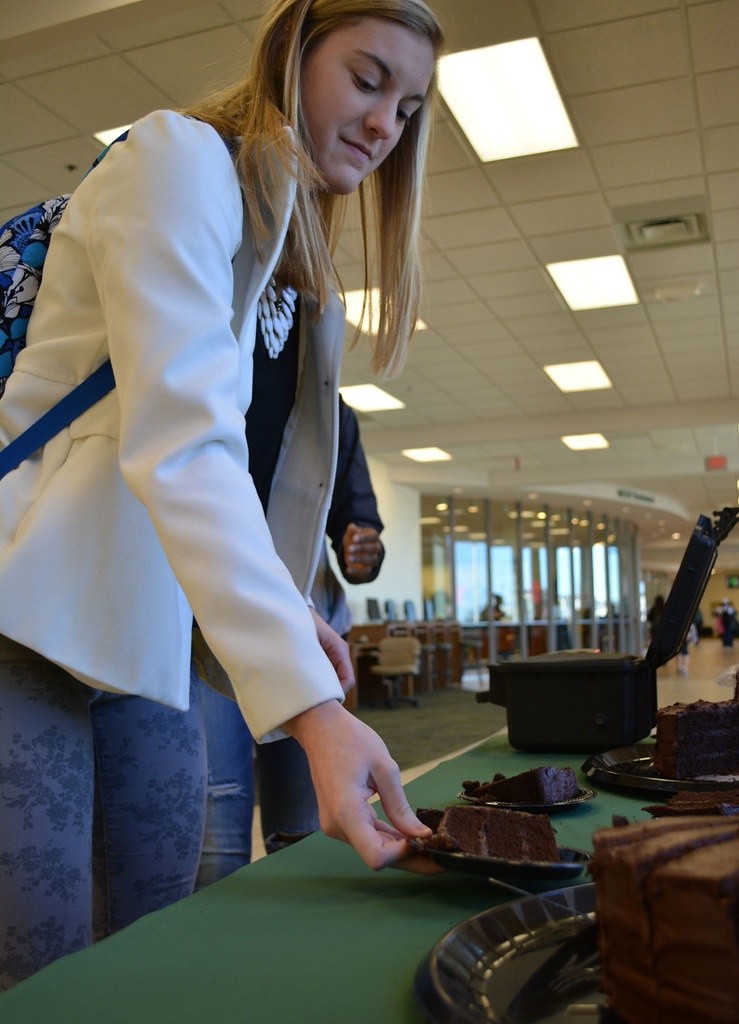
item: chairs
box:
[372,637,421,708]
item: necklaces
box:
[258,274,298,359]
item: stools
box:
[436,643,454,690]
[422,645,438,695]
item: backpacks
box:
[0,126,131,479]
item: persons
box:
[715,597,737,649]
[0,0,442,990]
[648,596,667,667]
[482,596,504,621]
[599,604,617,650]
[694,609,701,640]
[678,624,698,678]
[194,396,385,894]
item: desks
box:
[0,731,739,1024]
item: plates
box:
[430,883,624,1024]
[639,804,739,818]
[457,783,597,812]
[577,750,739,801]
[406,835,592,882]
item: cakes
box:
[660,789,739,809]
[592,810,739,1024]
[412,805,562,861]
[461,766,578,804]
[651,696,739,779]
